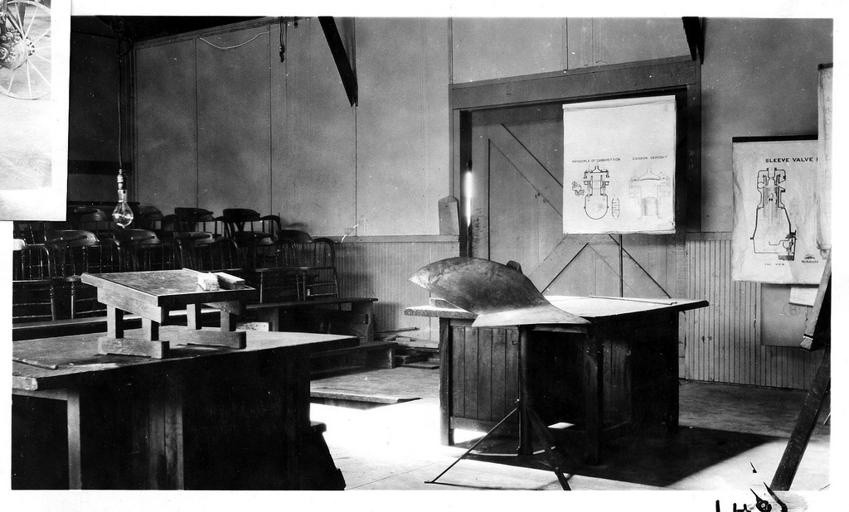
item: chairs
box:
[12,205,339,320]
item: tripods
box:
[424,326,577,490]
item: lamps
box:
[112,42,135,231]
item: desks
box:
[12,325,358,490]
[403,298,710,462]
